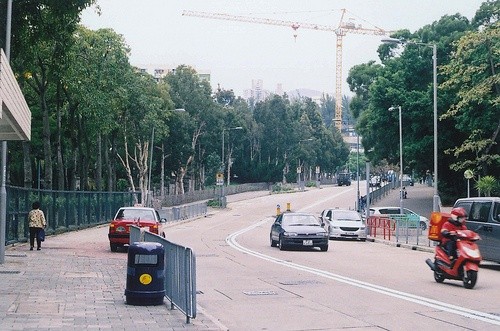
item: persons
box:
[441,207,483,265]
[28,201,47,250]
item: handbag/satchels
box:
[38,228,45,241]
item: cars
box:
[270,213,329,252]
[318,209,369,242]
[368,171,414,187]
[108,203,167,252]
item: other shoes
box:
[450,259,457,269]
[30,247,33,250]
[37,248,41,250]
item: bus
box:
[337,172,351,186]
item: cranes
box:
[179,7,399,134]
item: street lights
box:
[222,127,243,173]
[297,138,314,190]
[380,39,442,213]
[145,109,185,208]
[332,119,360,213]
[387,106,405,216]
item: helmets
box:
[451,207,468,222]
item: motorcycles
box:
[399,190,409,199]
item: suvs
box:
[362,207,430,230]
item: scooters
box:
[426,230,484,289]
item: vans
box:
[452,197,500,265]
